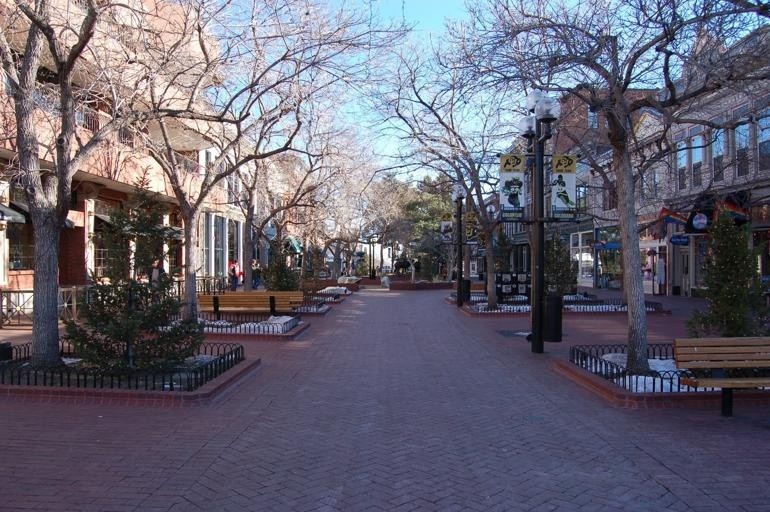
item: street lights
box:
[520,88,563,355]
[452,184,468,307]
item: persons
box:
[228,260,240,291]
[501,177,523,207]
[553,174,575,207]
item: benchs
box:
[226,291,304,316]
[670,337,770,417]
[196,295,291,317]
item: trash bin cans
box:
[541,296,563,342]
[460,280,470,302]
